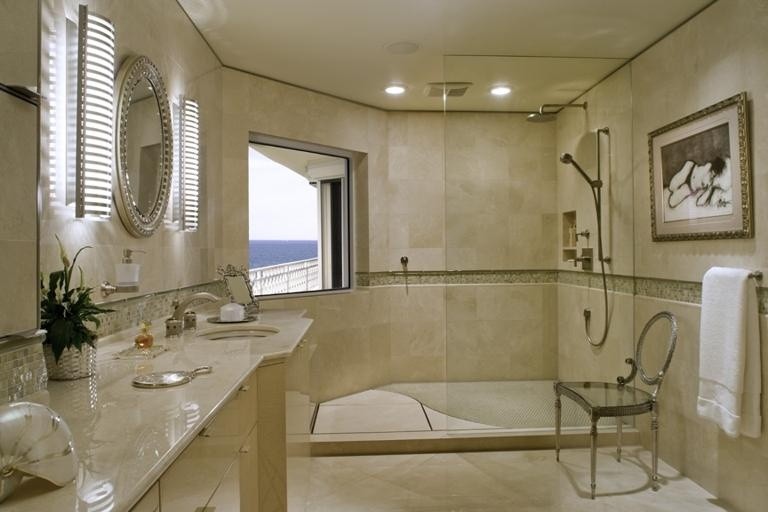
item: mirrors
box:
[113,51,177,239]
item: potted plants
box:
[27,233,117,381]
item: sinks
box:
[195,326,281,341]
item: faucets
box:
[173,292,222,319]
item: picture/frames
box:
[642,87,758,245]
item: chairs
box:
[546,310,682,500]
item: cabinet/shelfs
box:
[158,371,263,510]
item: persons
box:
[668,157,725,208]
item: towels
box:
[692,264,765,445]
